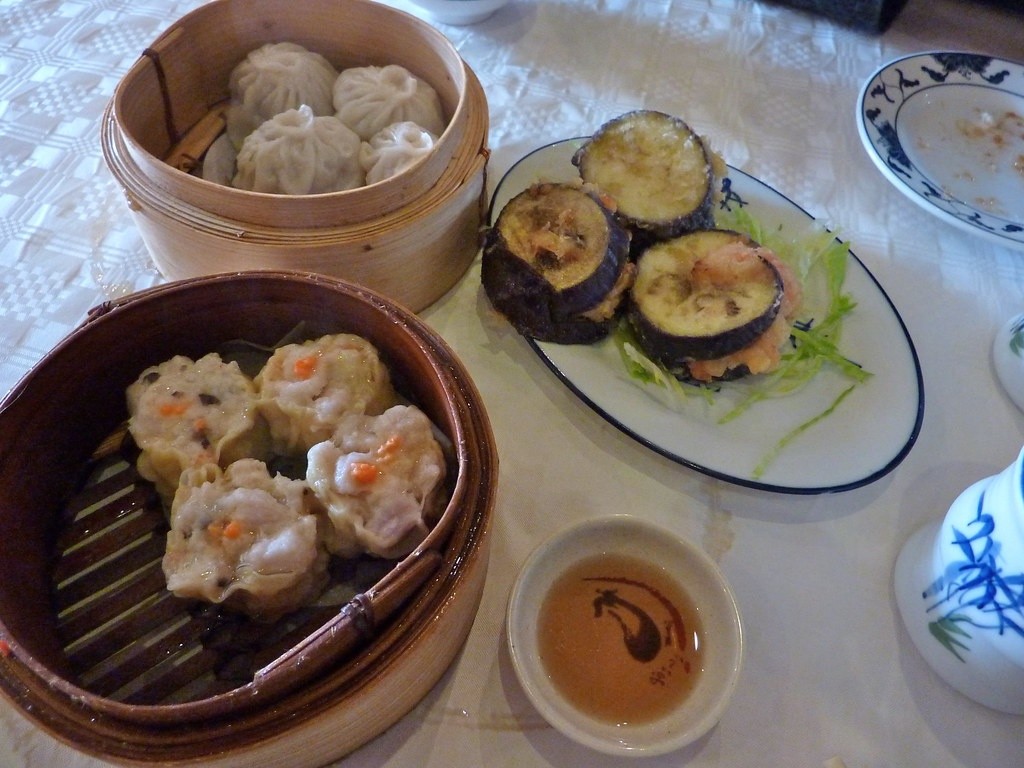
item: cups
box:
[892,447,1024,717]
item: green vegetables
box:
[607,209,875,478]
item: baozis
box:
[224,42,444,195]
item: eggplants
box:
[479,107,785,362]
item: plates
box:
[504,515,744,758]
[856,51,1024,253]
[484,133,926,490]
[994,312,1024,413]
